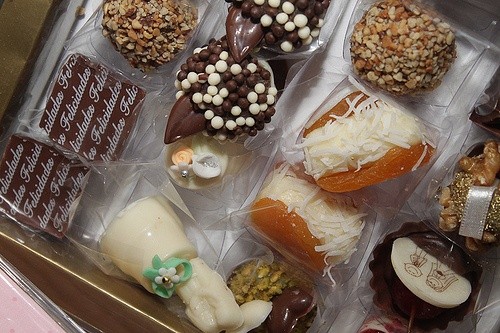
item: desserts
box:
[225,0,333,65]
[367,219,482,330]
[162,35,278,146]
[224,260,318,333]
[348,0,457,98]
[167,133,228,190]
[100,0,198,78]
